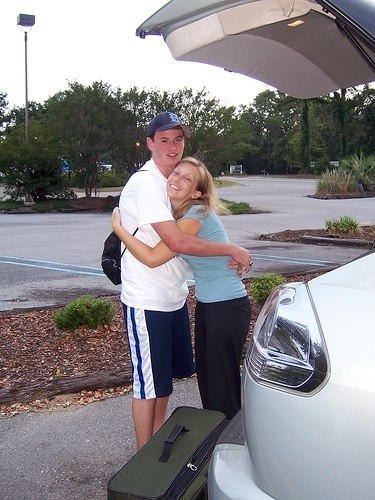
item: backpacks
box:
[101,170,149,285]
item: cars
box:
[207,246,375,500]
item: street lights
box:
[16,13,36,142]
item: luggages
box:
[107,405,229,500]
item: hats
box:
[147,112,191,138]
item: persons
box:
[111,158,251,422]
[119,112,254,450]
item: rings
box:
[250,260,253,265]
